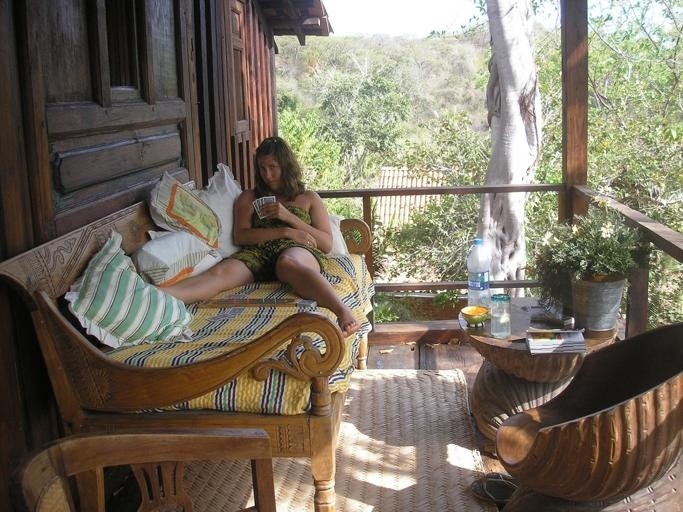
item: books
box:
[523,325,586,356]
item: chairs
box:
[6,424,277,512]
[498,321,682,512]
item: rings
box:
[306,240,310,245]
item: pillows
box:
[63,162,252,349]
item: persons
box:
[149,135,360,338]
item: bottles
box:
[467,236,490,309]
[490,294,511,340]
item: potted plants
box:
[526,200,642,331]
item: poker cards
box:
[252,196,275,219]
[296,300,316,307]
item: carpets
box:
[137,366,680,512]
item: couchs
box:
[0,180,374,512]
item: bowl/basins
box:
[460,304,488,328]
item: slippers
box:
[471,472,519,502]
